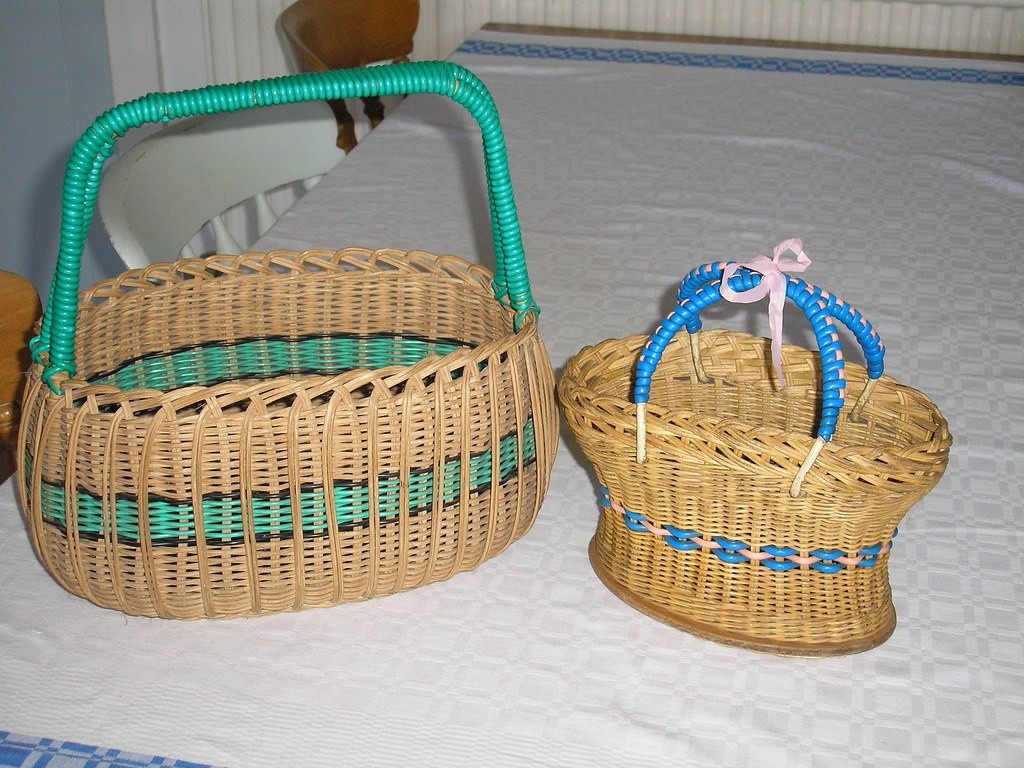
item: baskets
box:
[555,237,957,657]
[14,57,562,621]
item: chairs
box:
[282,0,420,154]
[98,99,372,284]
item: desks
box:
[0,22,1023,768]
